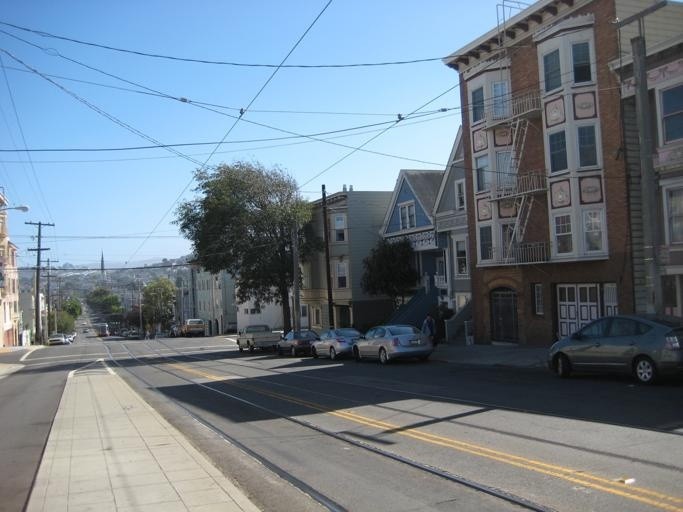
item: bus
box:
[96,322,110,337]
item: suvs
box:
[180,318,206,337]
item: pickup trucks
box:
[237,323,283,354]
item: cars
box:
[310,327,364,361]
[546,312,682,385]
[350,323,437,366]
[48,331,77,345]
[82,327,89,333]
[275,330,318,356]
[114,327,140,339]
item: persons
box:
[421,312,437,340]
